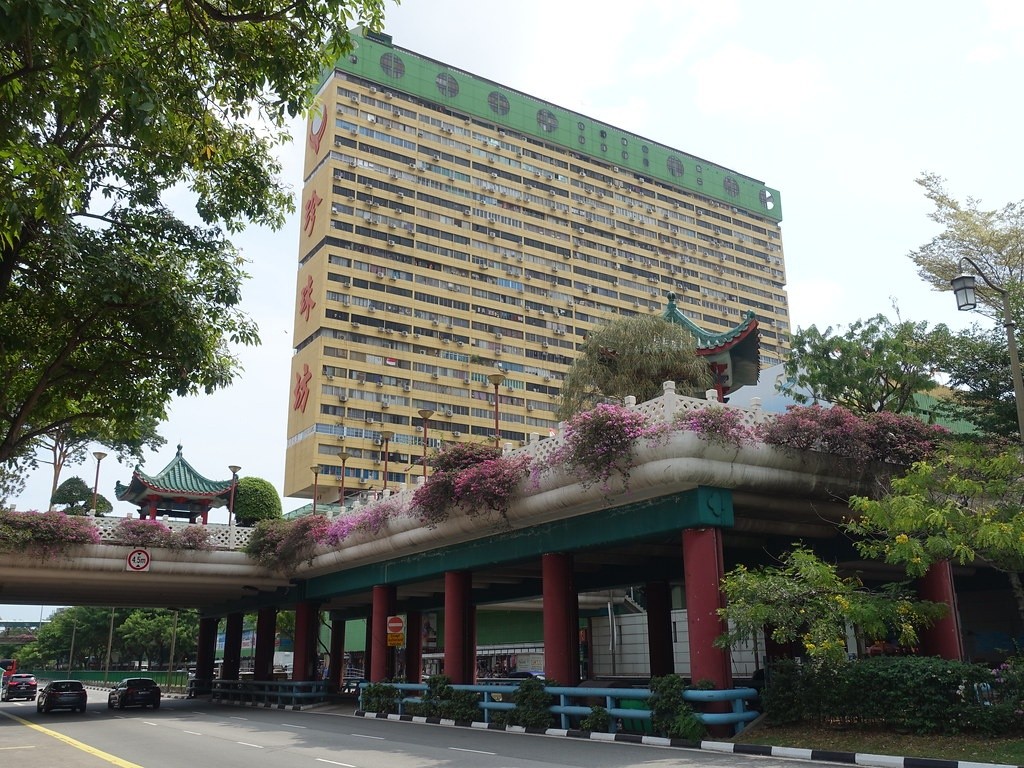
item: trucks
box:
[129,661,148,672]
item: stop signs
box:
[387,616,405,634]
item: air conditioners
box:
[329,85,786,500]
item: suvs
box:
[1,674,38,702]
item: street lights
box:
[336,452,351,507]
[227,466,242,526]
[309,466,324,516]
[417,409,434,482]
[486,373,506,450]
[379,430,394,490]
[92,452,108,509]
[950,254,1023,441]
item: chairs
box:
[619,698,655,733]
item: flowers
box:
[247,502,403,560]
[862,409,952,468]
[10,507,217,562]
[405,432,532,529]
[675,402,762,449]
[545,401,670,495]
[766,401,870,452]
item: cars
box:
[509,671,545,682]
[108,678,161,711]
[37,680,87,716]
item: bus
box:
[0,659,16,686]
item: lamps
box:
[241,585,278,597]
[166,606,206,616]
